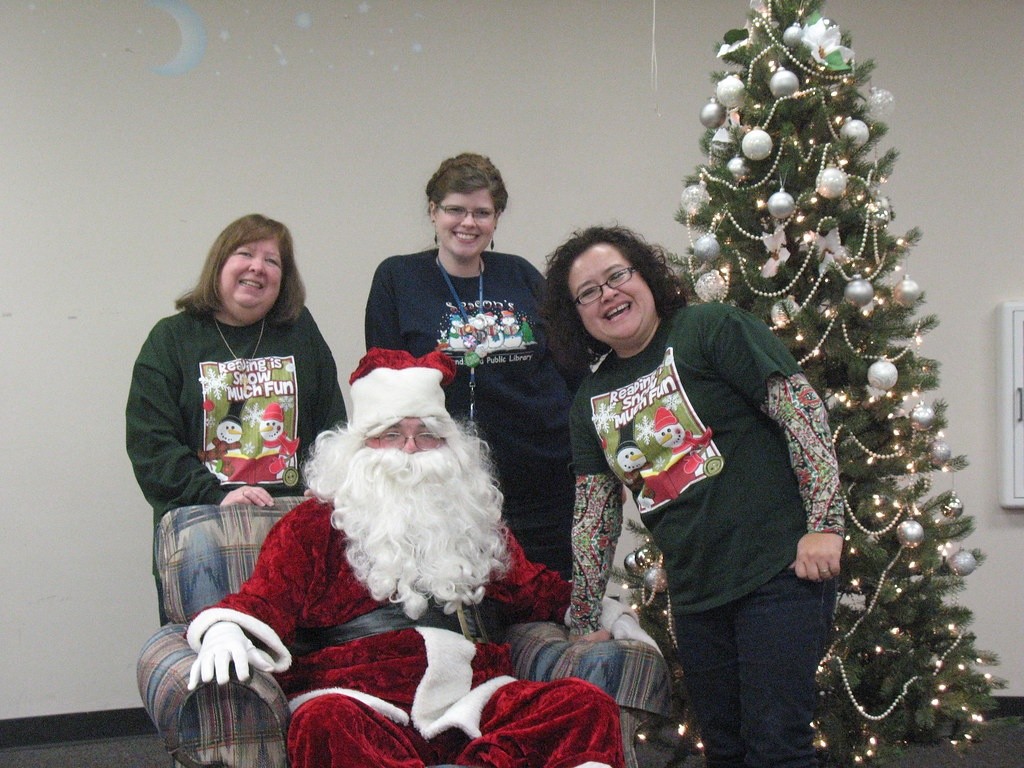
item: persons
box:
[542,217,845,768]
[126,214,348,626]
[364,153,595,586]
[184,348,667,768]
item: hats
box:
[350,346,456,415]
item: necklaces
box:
[213,314,265,390]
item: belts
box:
[289,597,494,654]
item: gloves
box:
[610,613,664,659]
[188,622,275,691]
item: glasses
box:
[573,263,638,306]
[436,202,496,220]
[372,431,442,453]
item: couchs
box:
[137,497,677,768]
